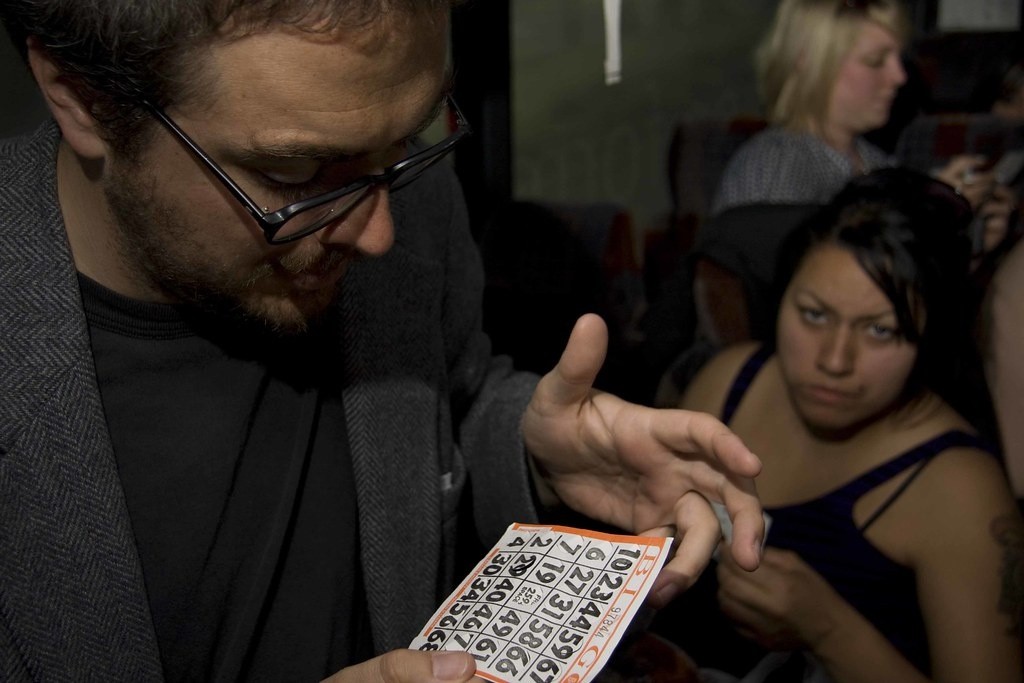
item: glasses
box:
[134,90,473,246]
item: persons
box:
[677,164,1024,683]
[703,0,1021,252]
[0,0,768,683]
